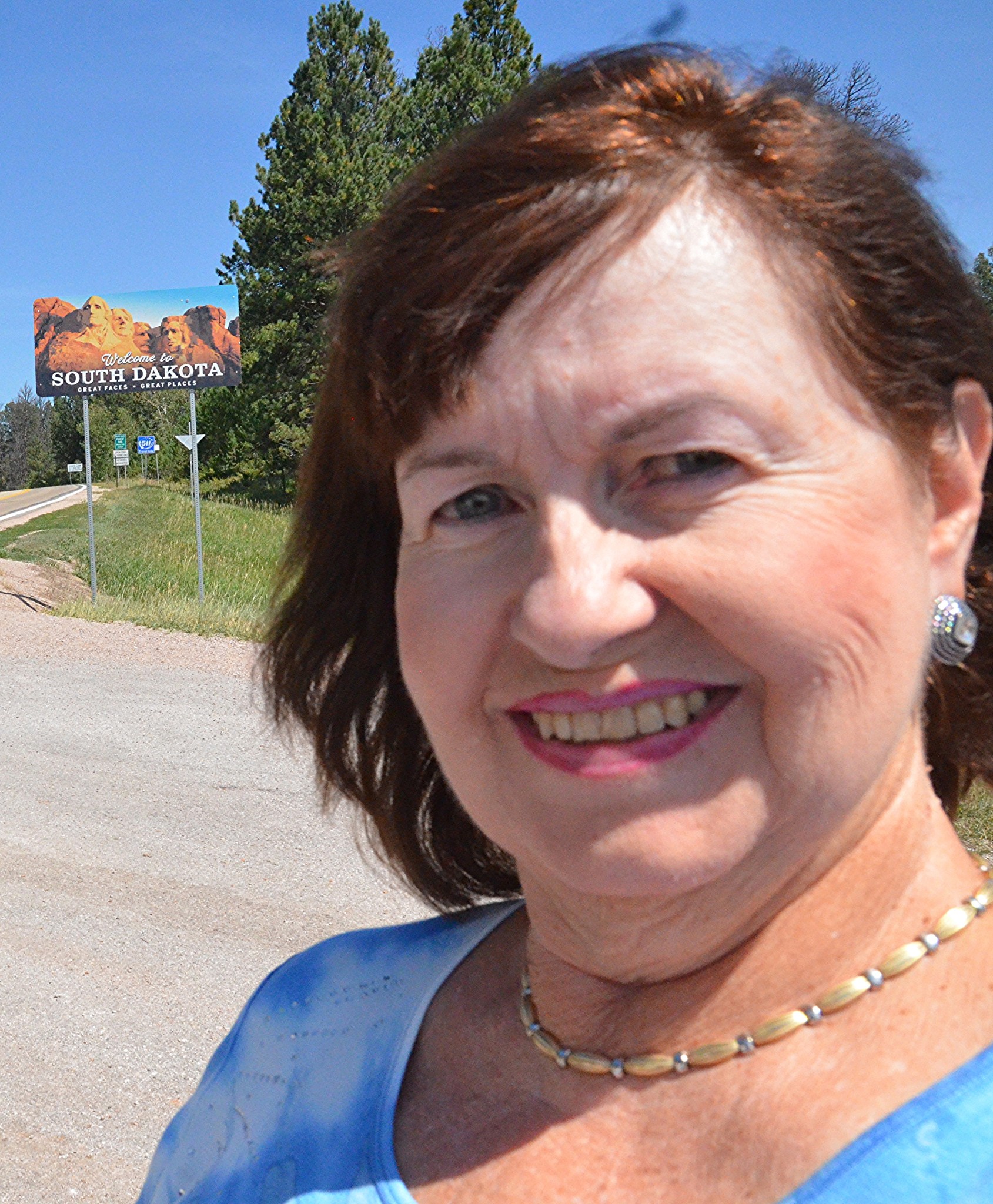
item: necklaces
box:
[519,852,993,1079]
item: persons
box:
[136,40,993,1204]
[162,321,183,352]
[80,295,150,352]
